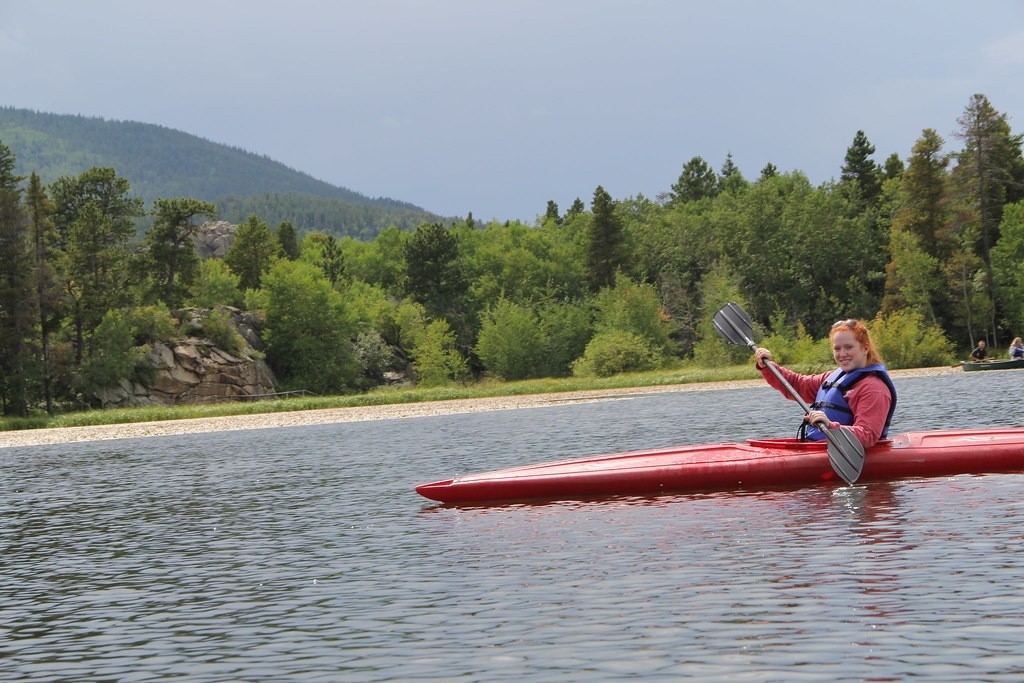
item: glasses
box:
[832,320,857,329]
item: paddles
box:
[711,302,866,484]
[951,357,995,367]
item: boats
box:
[960,359,1024,372]
[415,427,1024,510]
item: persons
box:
[969,341,988,364]
[756,320,897,448]
[1010,337,1024,360]
